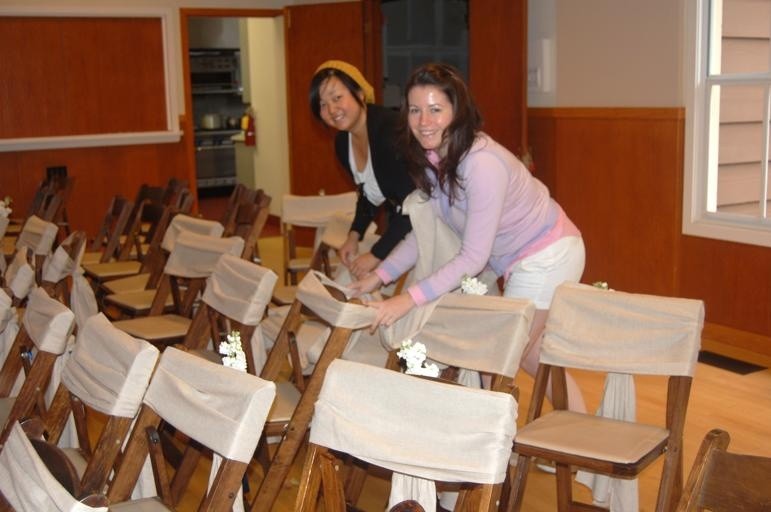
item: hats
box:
[314,59,375,104]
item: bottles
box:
[226,111,255,146]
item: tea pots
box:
[198,111,222,130]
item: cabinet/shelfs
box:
[189,48,241,188]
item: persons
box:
[307,59,502,387]
[339,62,593,474]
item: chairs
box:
[292,355,520,511]
[346,290,536,508]
[248,270,365,512]
[73,347,284,512]
[2,166,539,450]
[504,278,731,512]
[168,257,281,508]
[0,312,161,512]
